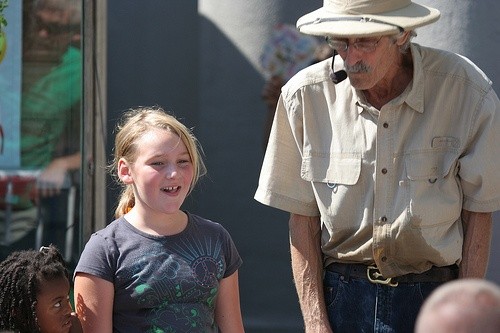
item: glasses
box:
[324,36,383,51]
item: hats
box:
[296,0,441,38]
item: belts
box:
[328,263,458,283]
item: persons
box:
[414,277,500,332]
[22,0,84,204]
[252,0,500,333]
[0,244,73,333]
[73,106,244,333]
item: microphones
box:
[330,49,348,85]
